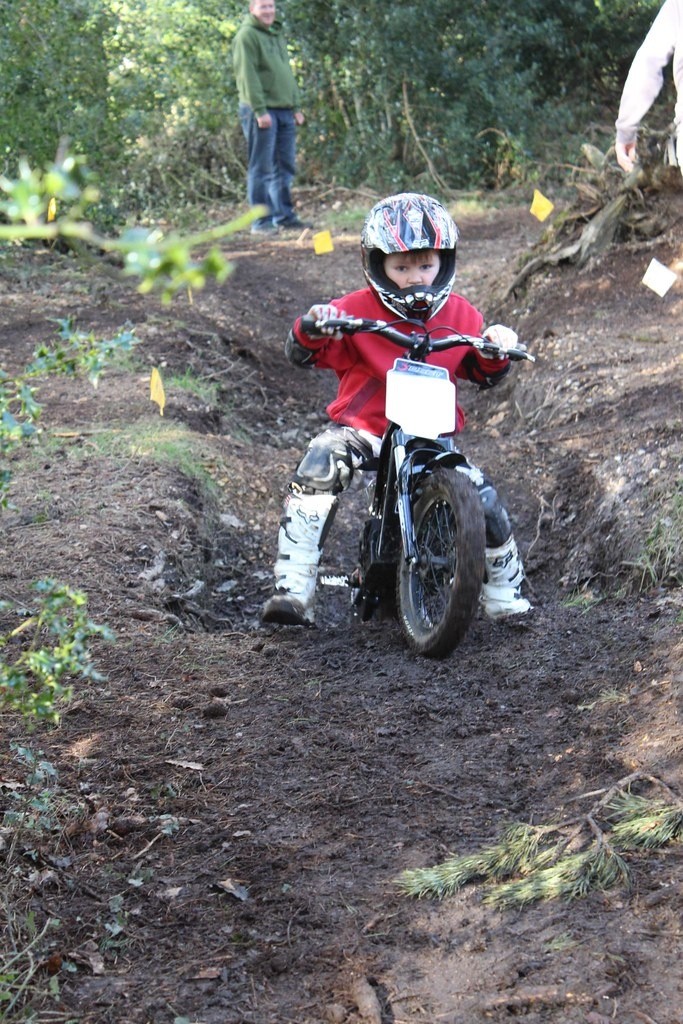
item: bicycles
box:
[289,309,542,658]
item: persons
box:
[232,0,314,237]
[614,0,683,178]
[262,193,531,625]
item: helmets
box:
[361,192,461,323]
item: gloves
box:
[308,303,347,340]
[479,324,519,361]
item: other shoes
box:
[251,227,279,235]
[281,219,314,230]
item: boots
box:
[478,534,533,618]
[260,493,340,627]
